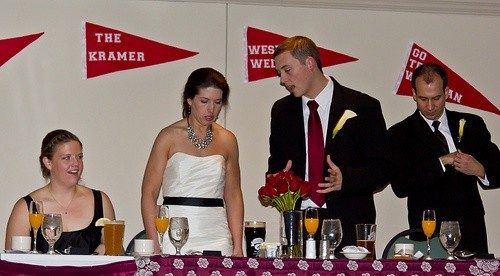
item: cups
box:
[261,243,280,259]
[304,209,319,238]
[245,221,265,257]
[134,239,154,256]
[12,237,31,252]
[394,244,414,258]
[104,220,125,256]
[355,224,376,258]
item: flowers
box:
[257,171,311,210]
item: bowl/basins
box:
[340,251,371,259]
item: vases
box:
[278,209,303,259]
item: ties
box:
[431,120,450,156]
[306,100,326,208]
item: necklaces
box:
[187,118,213,149]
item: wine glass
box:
[28,201,43,253]
[41,214,63,254]
[421,210,436,260]
[439,221,461,260]
[168,216,189,255]
[321,219,342,259]
[156,205,170,256]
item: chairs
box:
[126,230,145,258]
[382,230,448,259]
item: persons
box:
[141,68,244,256]
[266,35,389,258]
[387,63,500,254]
[5,129,116,255]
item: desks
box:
[0,252,500,276]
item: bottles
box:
[319,235,330,260]
[306,237,316,258]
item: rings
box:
[332,178,336,182]
[331,182,334,187]
